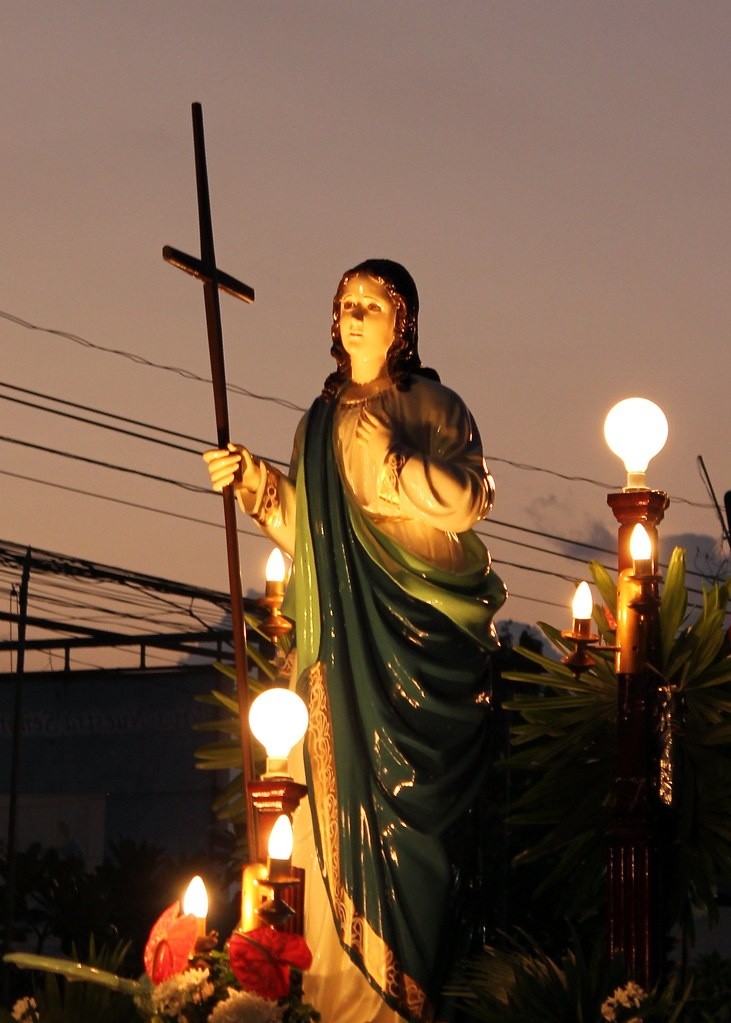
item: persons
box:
[203,258,507,1023]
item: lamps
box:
[570,397,667,640]
[175,542,304,962]
